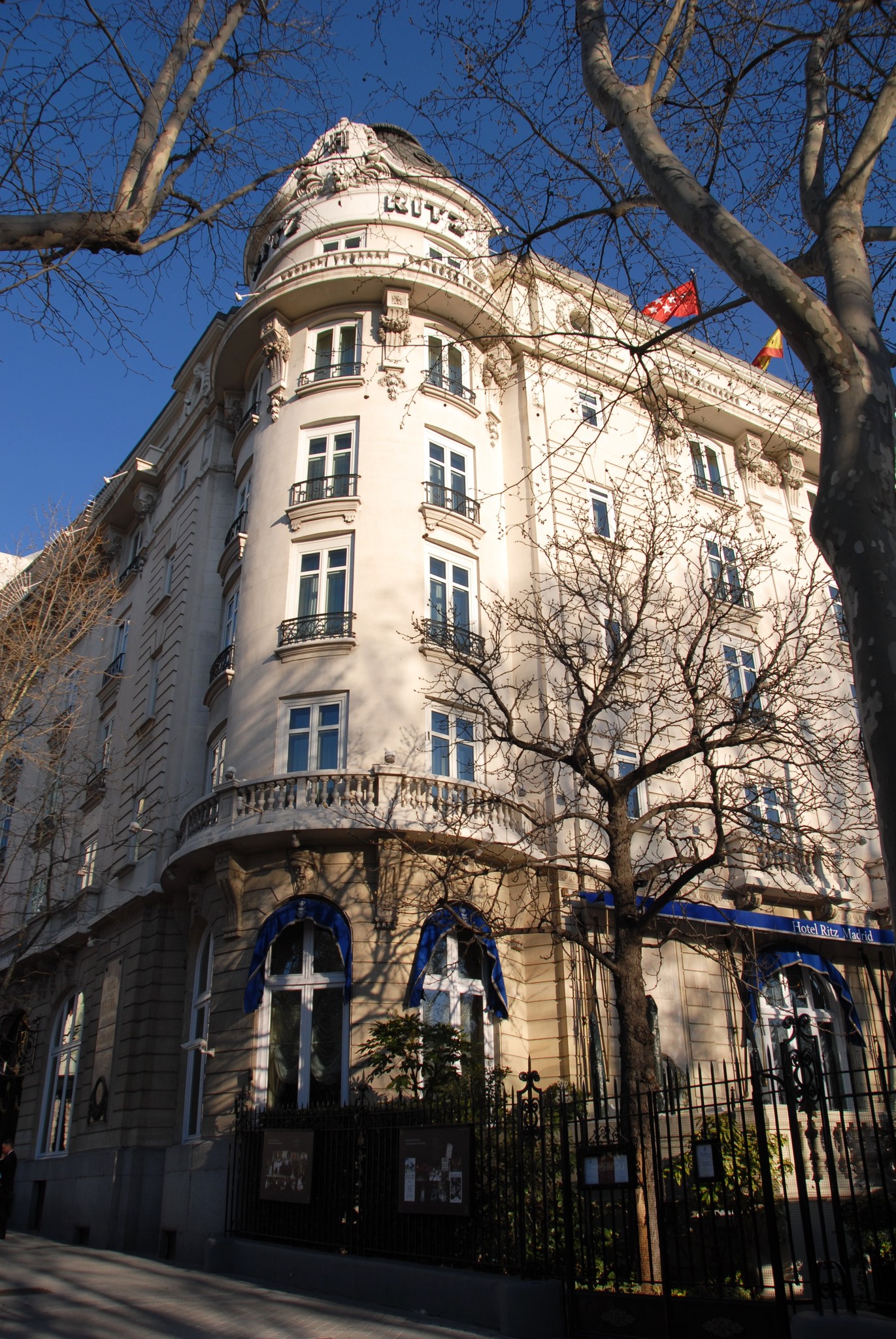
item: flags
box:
[640,280,699,329]
[749,327,781,372]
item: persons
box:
[0,1139,18,1240]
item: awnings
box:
[579,890,896,959]
